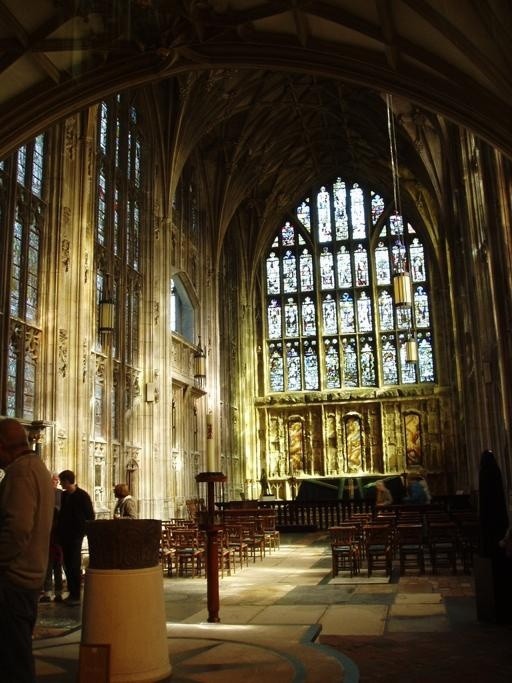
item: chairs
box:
[161,501,280,578]
[328,503,478,579]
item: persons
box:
[112,483,138,520]
[58,469,96,605]
[374,479,394,506]
[39,475,65,603]
[0,419,56,682]
[400,473,432,503]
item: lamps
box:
[195,334,206,378]
[384,92,414,308]
[98,274,115,333]
[385,91,421,365]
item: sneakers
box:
[40,596,80,606]
